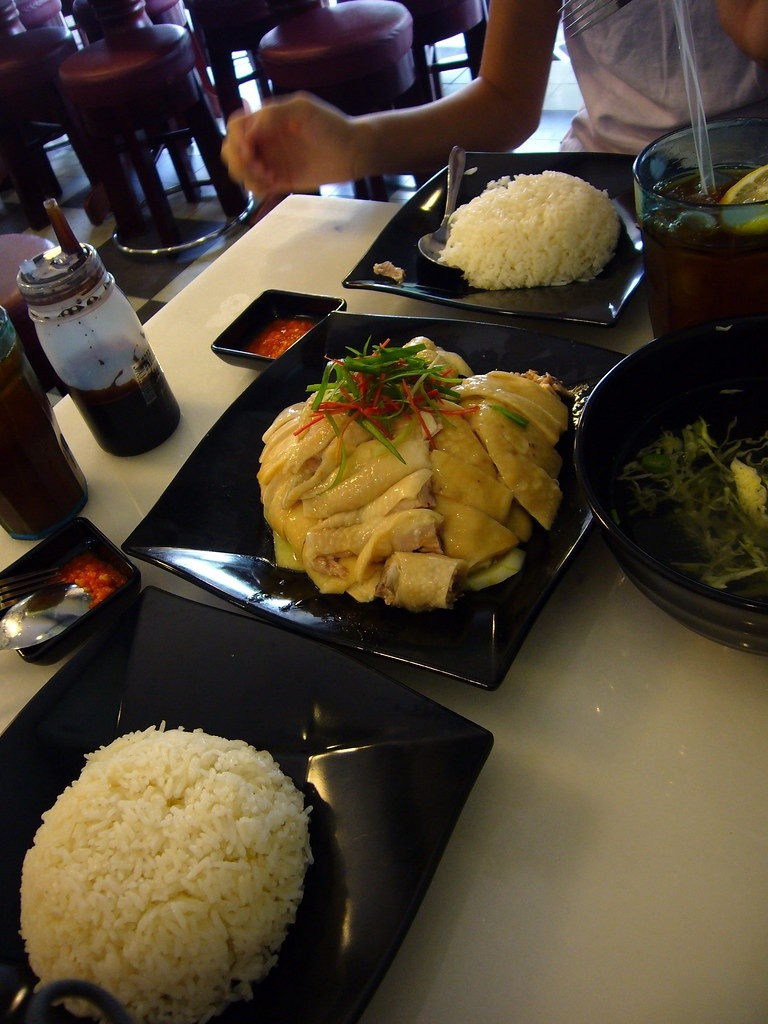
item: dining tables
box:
[0,192,768,1024]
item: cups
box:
[633,119,768,342]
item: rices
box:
[437,168,620,290]
[18,721,315,1024]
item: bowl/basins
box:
[210,290,348,370]
[575,338,768,656]
[0,516,143,667]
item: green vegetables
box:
[610,414,768,602]
[291,340,527,496]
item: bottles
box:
[16,194,181,457]
[0,305,88,540]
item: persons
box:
[221,0,768,199]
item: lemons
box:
[717,163,768,235]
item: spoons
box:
[0,584,93,650]
[418,146,469,270]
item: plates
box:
[343,151,650,328]
[119,306,632,692]
[0,587,493,1024]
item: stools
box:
[0,0,497,398]
